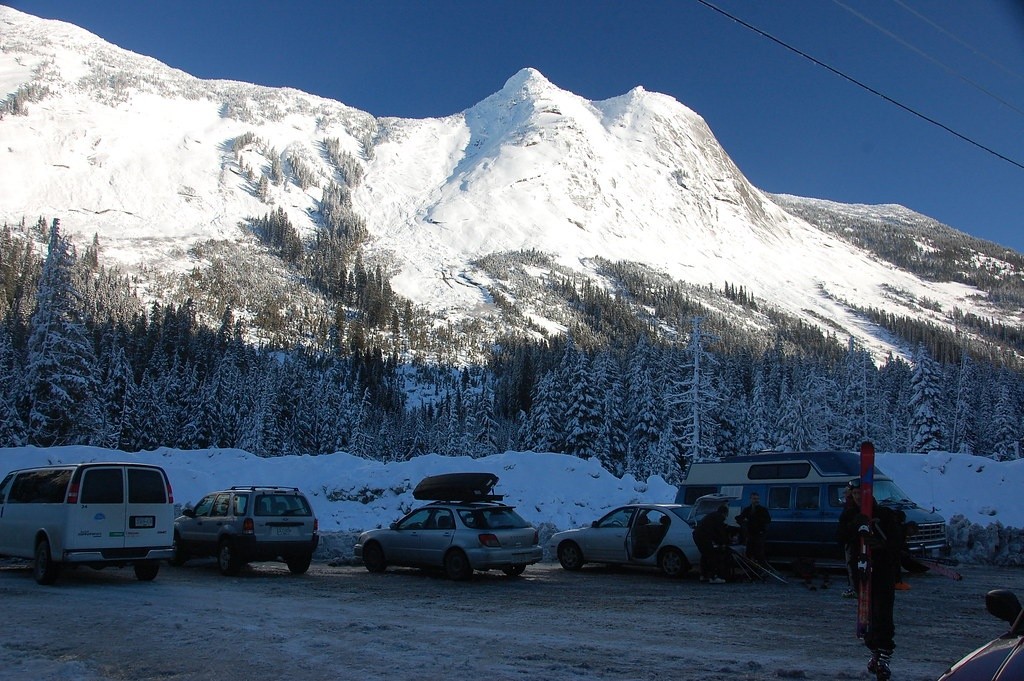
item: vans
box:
[0,462,174,585]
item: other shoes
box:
[842,588,855,597]
[709,575,726,584]
[895,582,913,590]
[700,572,713,581]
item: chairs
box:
[259,502,287,514]
[634,515,670,552]
[438,516,451,529]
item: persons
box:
[693,506,729,583]
[737,493,771,582]
[835,480,919,598]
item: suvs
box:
[173,484,320,577]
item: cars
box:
[548,494,746,579]
[938,588,1024,681]
[354,501,542,580]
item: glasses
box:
[847,479,860,490]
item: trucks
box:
[675,450,951,577]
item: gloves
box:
[854,520,874,536]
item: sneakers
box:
[867,650,877,673]
[875,648,893,679]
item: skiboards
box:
[727,542,793,586]
[900,550,963,581]
[857,441,875,638]
